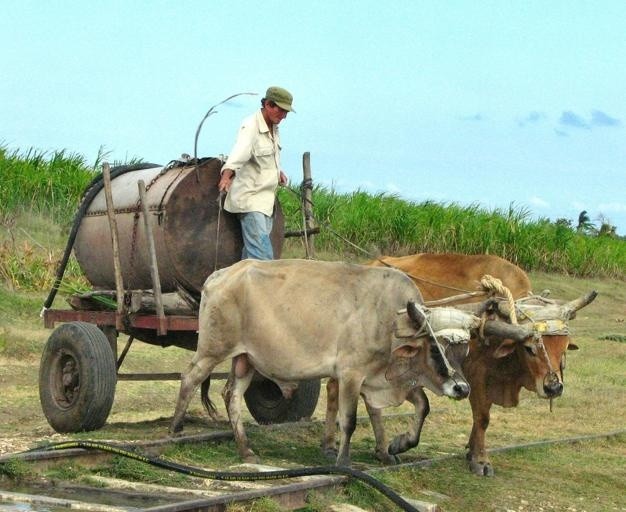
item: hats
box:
[265,86,296,114]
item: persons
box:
[216,86,297,262]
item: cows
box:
[167,257,532,469]
[319,251,598,481]
